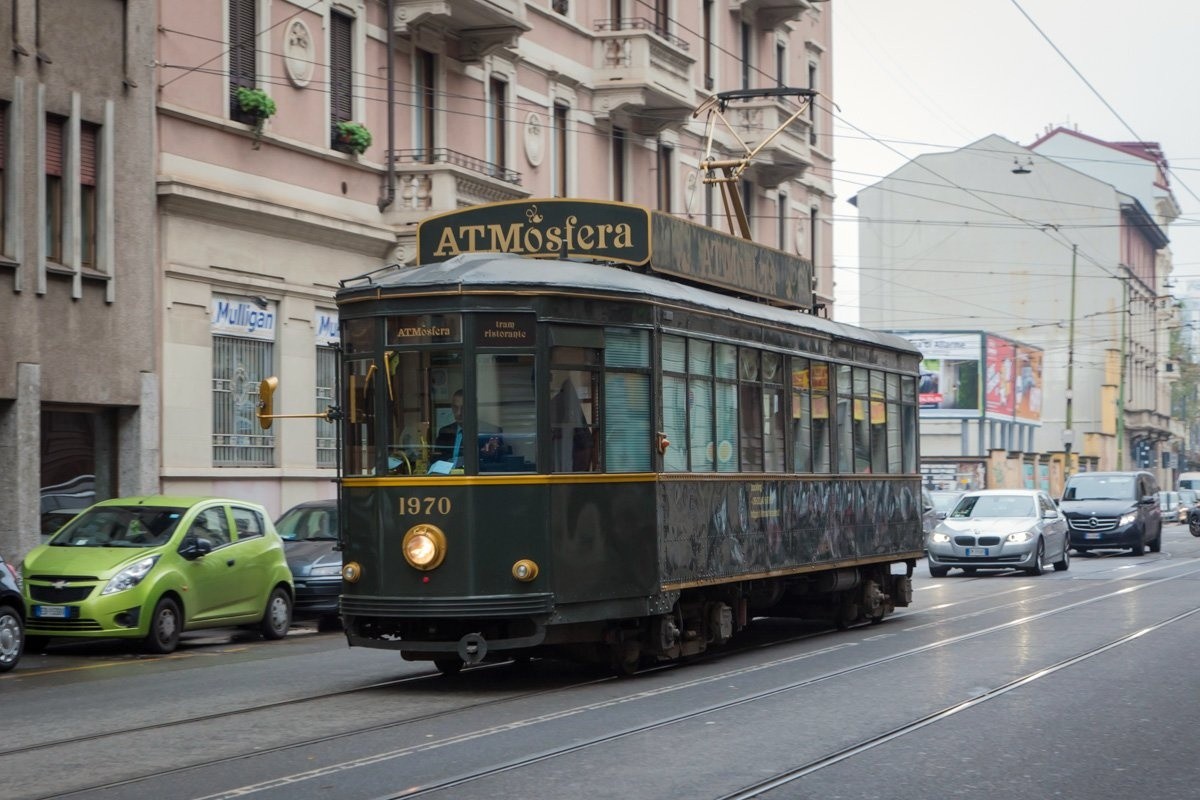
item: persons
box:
[433,390,503,463]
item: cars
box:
[1187,503,1200,538]
[15,498,292,655]
[919,487,1070,576]
[272,500,343,634]
[1158,491,1177,524]
[0,555,26,672]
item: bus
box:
[254,88,925,681]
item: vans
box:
[1177,472,1200,506]
[1058,471,1162,555]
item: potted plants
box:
[332,117,374,157]
[233,83,276,123]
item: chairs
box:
[224,517,253,538]
